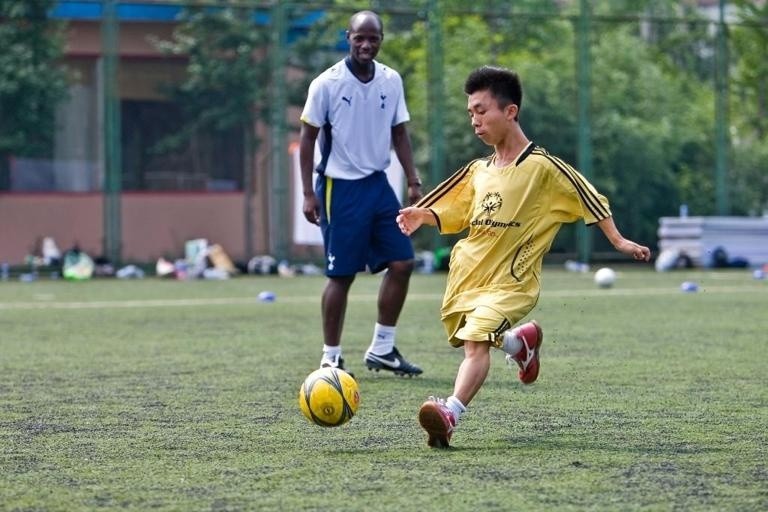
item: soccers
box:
[299,368,360,428]
[595,267,617,287]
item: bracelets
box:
[409,176,422,186]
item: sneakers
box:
[320,355,354,378]
[505,320,543,383]
[364,347,423,377]
[419,395,456,448]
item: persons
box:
[300,9,423,379]
[397,65,652,451]
